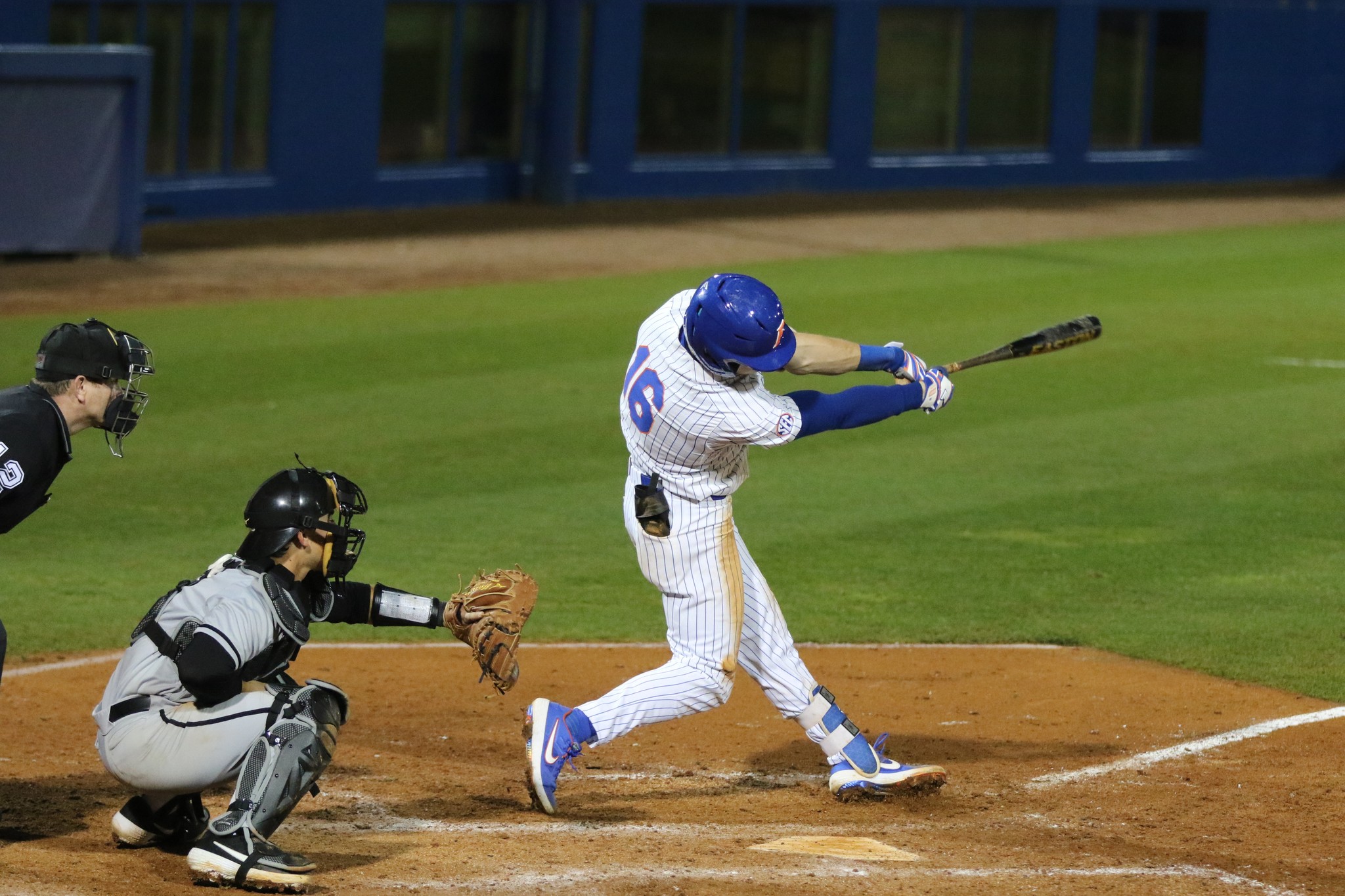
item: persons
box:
[529,273,956,815]
[93,467,537,896]
[0,317,156,688]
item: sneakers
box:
[111,795,210,847]
[828,732,946,799]
[526,697,586,816]
[186,829,314,893]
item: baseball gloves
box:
[443,569,539,695]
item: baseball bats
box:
[930,315,1103,376]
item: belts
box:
[108,694,151,723]
[629,463,726,500]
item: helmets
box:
[236,468,335,562]
[683,274,797,378]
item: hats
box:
[34,322,129,382]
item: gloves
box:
[920,369,954,412]
[883,341,926,383]
